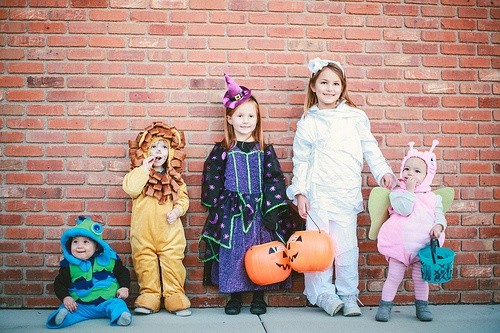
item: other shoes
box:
[250,302,267,313]
[416,300,433,321]
[225,301,242,314]
[340,294,364,315]
[375,300,393,321]
[134,307,151,313]
[315,293,344,316]
[176,308,191,316]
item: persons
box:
[122,121,192,317]
[287,58,397,316]
[376,140,446,321]
[199,72,304,315]
[46,216,131,328]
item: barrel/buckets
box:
[419,237,455,283]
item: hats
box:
[399,138,439,192]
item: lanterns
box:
[286,230,334,272]
[244,241,291,286]
